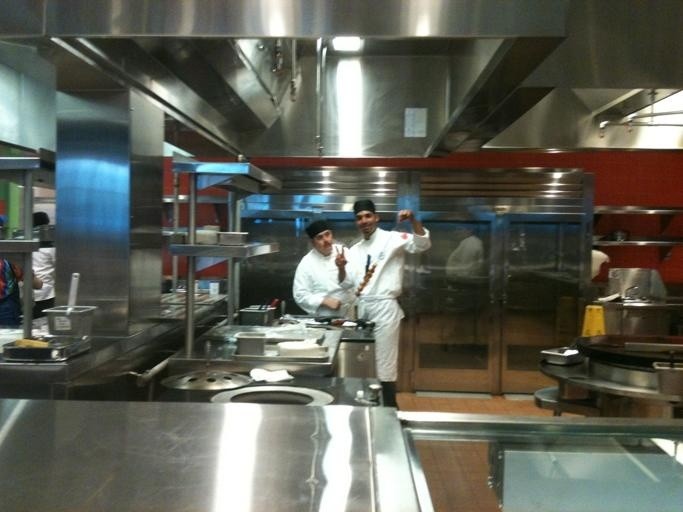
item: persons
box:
[29,212,56,321]
[0,214,43,327]
[335,200,432,411]
[292,219,356,320]
[444,227,487,341]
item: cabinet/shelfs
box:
[406,169,596,393]
[0,152,55,334]
[169,161,286,348]
[239,163,405,394]
[161,194,229,297]
[589,203,683,255]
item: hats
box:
[354,200,375,215]
[306,221,332,238]
